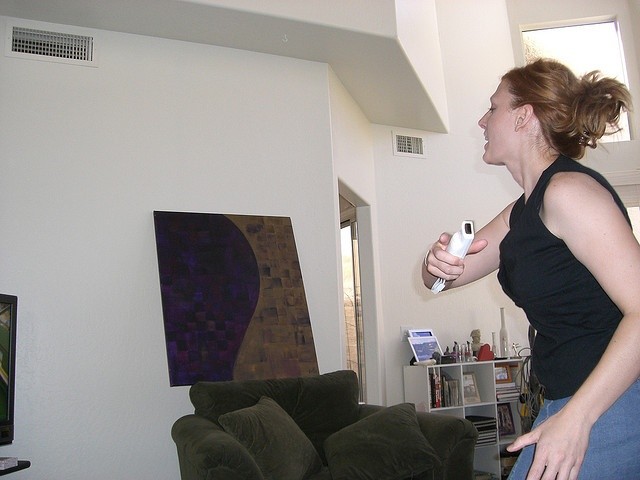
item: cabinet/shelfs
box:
[402,358,526,479]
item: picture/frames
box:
[407,336,444,363]
[494,363,513,384]
[407,329,434,336]
[497,402,517,437]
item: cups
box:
[455,344,465,363]
[465,345,473,363]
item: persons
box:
[470,329,484,350]
[420,58,640,480]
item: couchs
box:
[172,367,479,478]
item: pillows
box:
[320,401,444,479]
[216,396,323,479]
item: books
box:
[494,382,520,401]
[464,415,497,447]
[428,369,460,408]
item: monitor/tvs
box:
[0,294,17,447]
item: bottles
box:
[499,308,509,357]
